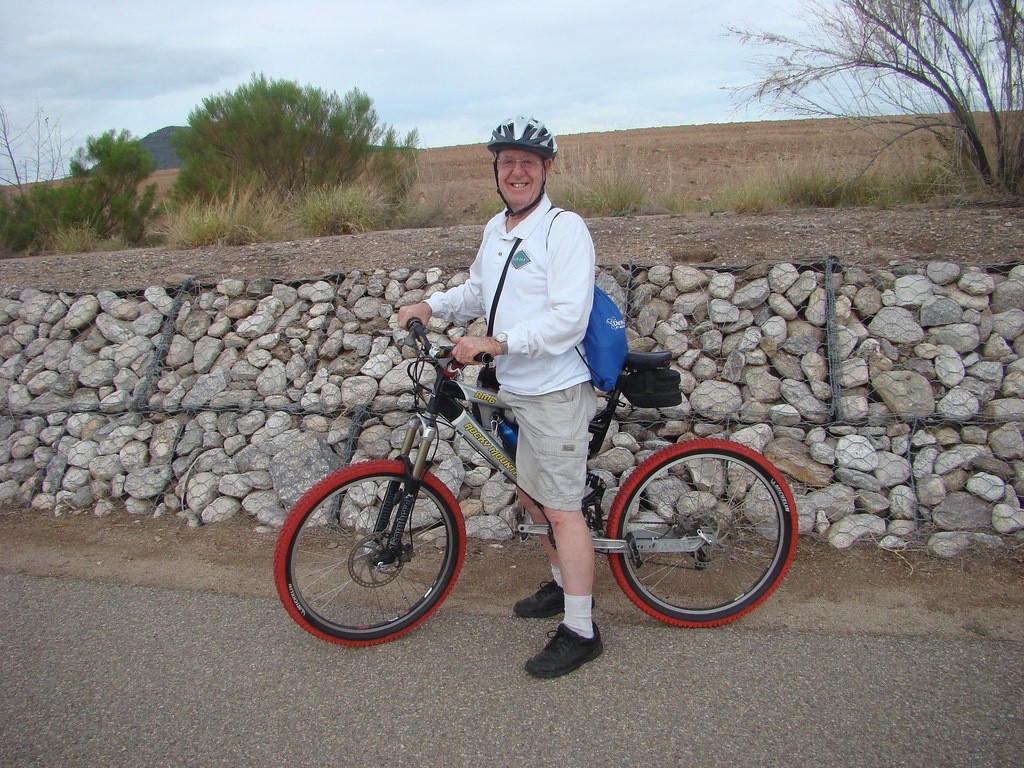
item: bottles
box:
[490,411,519,453]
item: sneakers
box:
[514,579,596,618]
[525,623,603,678]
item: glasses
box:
[495,157,545,170]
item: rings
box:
[461,355,466,358]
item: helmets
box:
[486,117,558,161]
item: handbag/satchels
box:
[472,365,501,434]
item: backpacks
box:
[545,210,627,393]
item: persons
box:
[399,116,603,678]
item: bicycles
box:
[273,316,799,647]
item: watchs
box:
[495,332,509,356]
